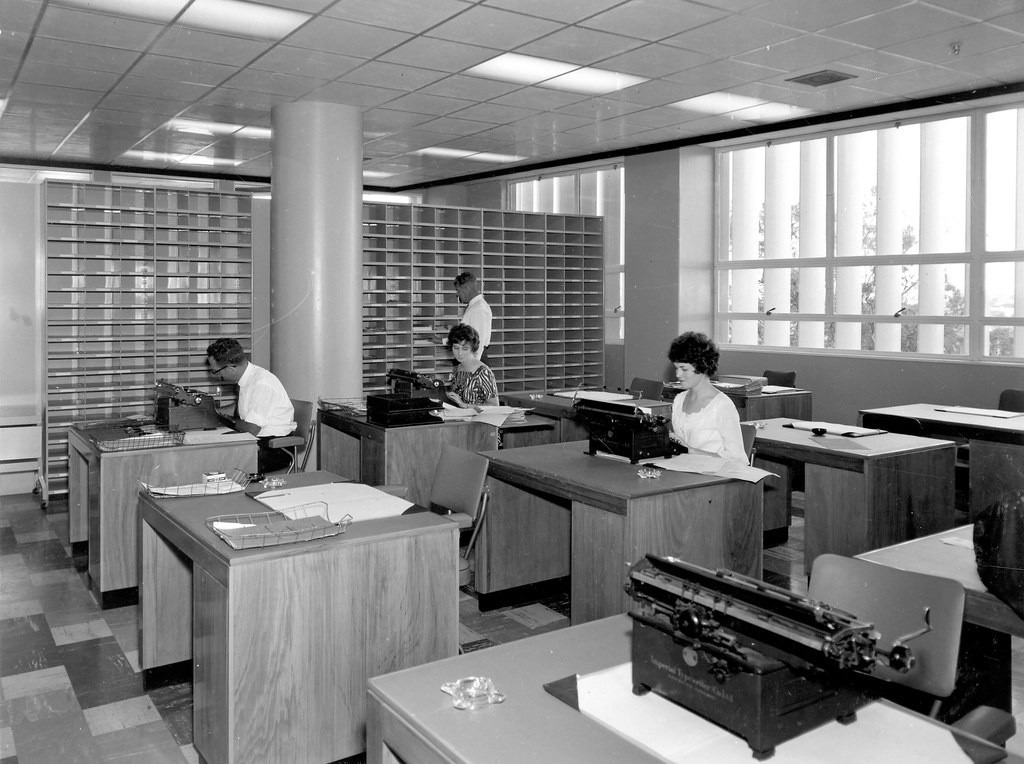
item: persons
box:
[424,272,493,369]
[201,338,298,483]
[668,330,749,466]
[446,322,501,448]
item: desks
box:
[851,521,1024,724]
[500,392,675,443]
[364,613,1024,764]
[664,382,812,421]
[318,408,497,520]
[137,469,460,764]
[67,420,260,611]
[475,439,765,627]
[858,403,1024,521]
[739,418,955,575]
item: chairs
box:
[628,378,665,400]
[762,369,796,388]
[810,554,966,720]
[373,443,490,587]
[269,398,317,473]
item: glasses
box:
[212,366,236,375]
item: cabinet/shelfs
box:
[36,178,608,510]
